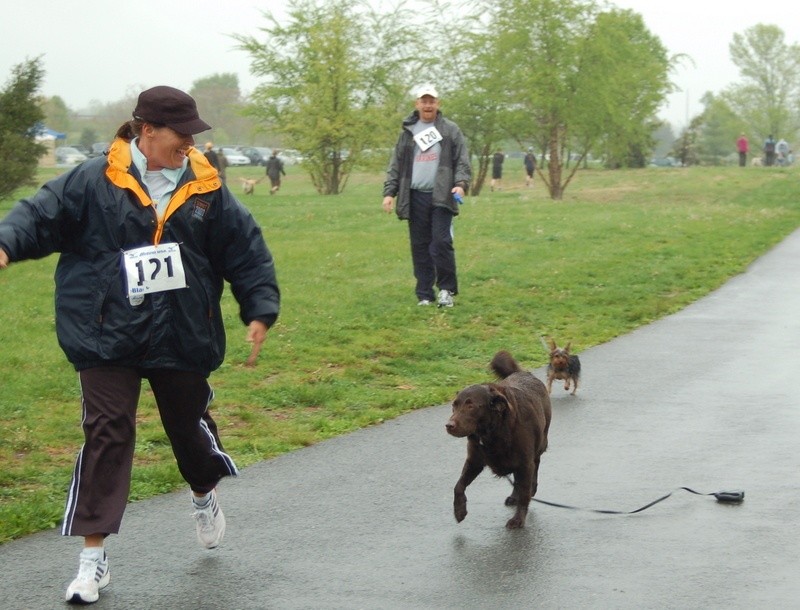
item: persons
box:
[382,85,471,308]
[523,147,538,187]
[204,143,286,196]
[735,132,794,169]
[0,85,282,606]
[489,148,505,191]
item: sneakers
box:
[189,486,225,550]
[436,289,453,307]
[417,299,433,306]
[65,549,110,604]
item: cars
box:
[243,147,309,166]
[194,144,251,168]
[57,143,111,165]
[329,147,395,162]
[501,151,588,162]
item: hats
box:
[416,85,438,98]
[132,86,212,135]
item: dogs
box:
[548,336,582,396]
[240,177,254,194]
[445,350,551,531]
[751,157,762,166]
